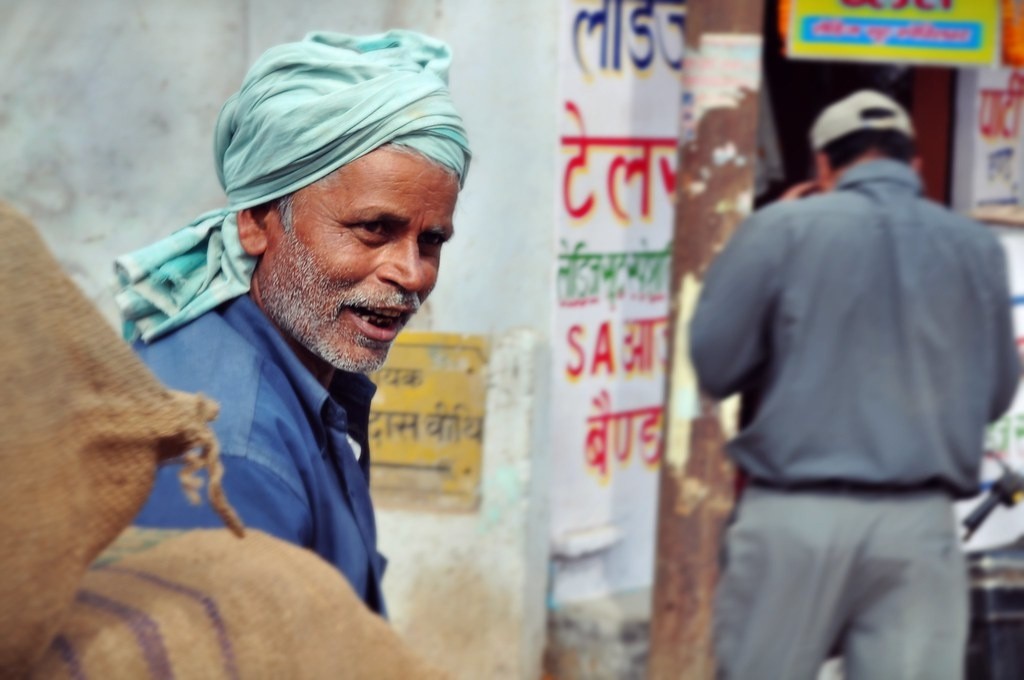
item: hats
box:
[810,90,915,151]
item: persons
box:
[687,89,1019,680]
[112,26,472,626]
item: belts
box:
[745,478,956,493]
